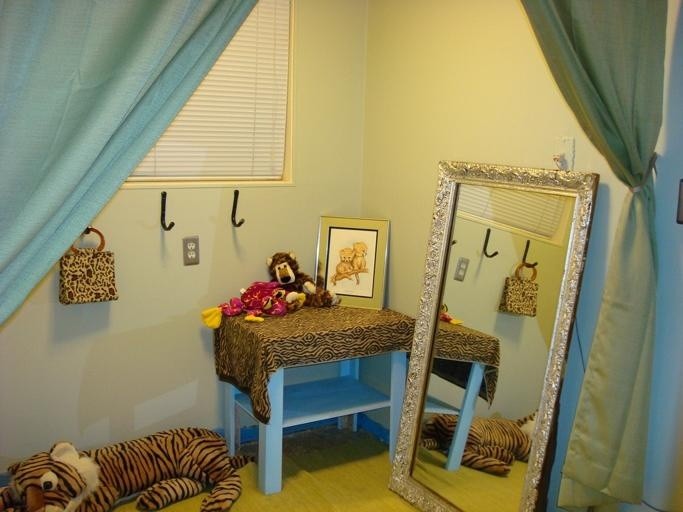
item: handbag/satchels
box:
[498,263,539,317]
[59,228,119,305]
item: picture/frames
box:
[313,215,391,311]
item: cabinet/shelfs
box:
[422,320,500,472]
[212,316,416,494]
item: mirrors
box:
[385,157,600,511]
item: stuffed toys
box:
[264,250,338,308]
[0,427,256,511]
[421,410,542,479]
[199,280,288,329]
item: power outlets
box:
[452,256,470,283]
[182,234,199,266]
